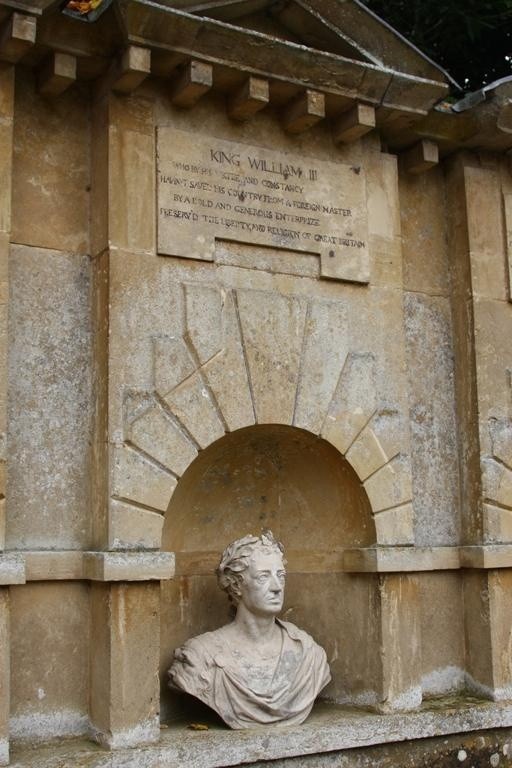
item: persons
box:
[163,531,332,730]
